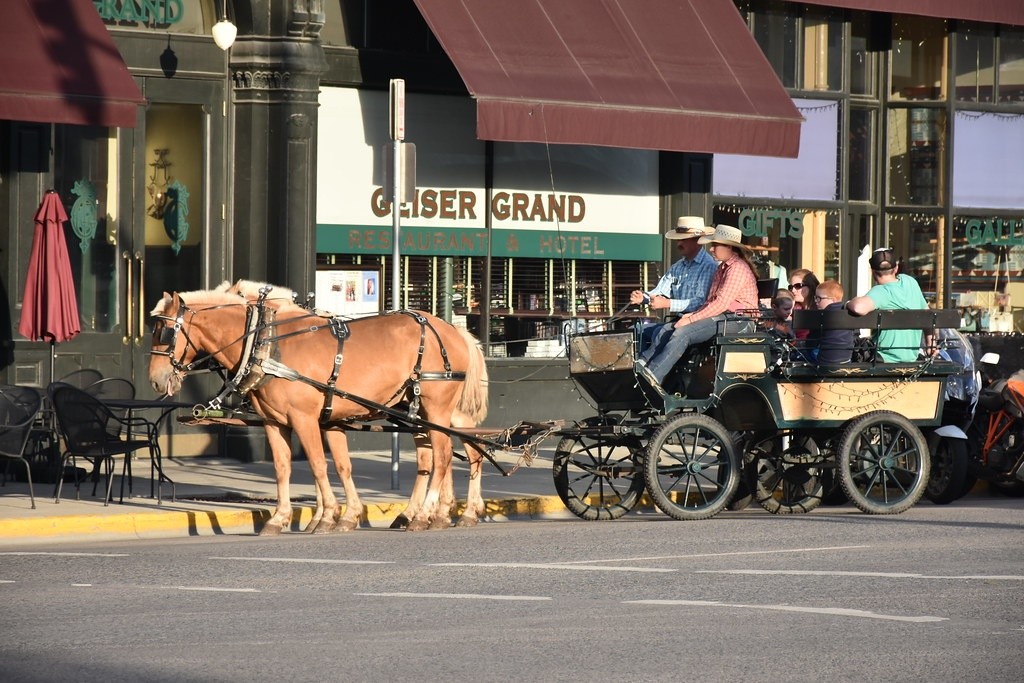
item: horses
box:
[148,278,489,538]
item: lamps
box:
[212,0,237,52]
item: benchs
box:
[664,276,965,402]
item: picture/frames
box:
[316,263,383,319]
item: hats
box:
[664,213,716,239]
[697,223,749,251]
[869,247,896,270]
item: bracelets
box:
[689,316,693,324]
[844,300,850,309]
[641,292,650,306]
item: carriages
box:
[146,280,965,541]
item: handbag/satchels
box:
[990,308,1013,333]
[994,294,1009,306]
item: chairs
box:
[0,371,176,510]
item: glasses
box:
[813,295,836,301]
[788,283,813,290]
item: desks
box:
[98,398,196,499]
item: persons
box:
[761,286,796,350]
[629,216,718,401]
[896,254,909,273]
[367,279,374,295]
[804,278,854,368]
[784,269,821,363]
[634,224,759,390]
[844,245,940,363]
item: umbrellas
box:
[18,188,82,383]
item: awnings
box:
[1,0,149,132]
[412,0,804,162]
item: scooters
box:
[925,324,1024,505]
